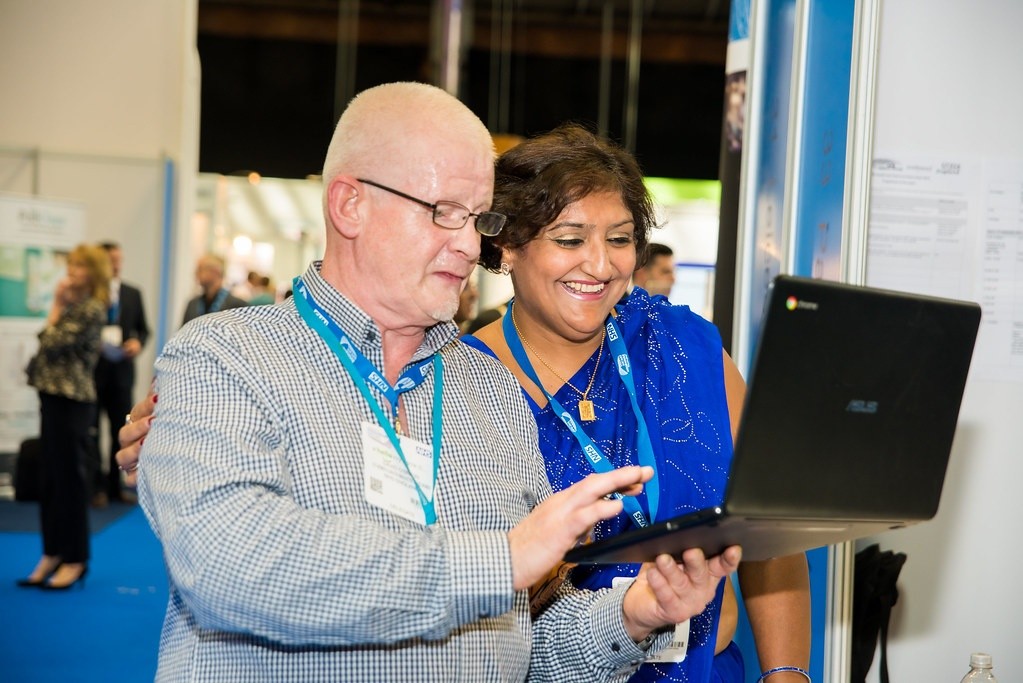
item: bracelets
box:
[756,666,811,683]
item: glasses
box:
[355,178,507,237]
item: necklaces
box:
[512,303,606,421]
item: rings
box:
[126,414,133,424]
[119,462,136,470]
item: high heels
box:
[17,555,63,588]
[38,561,88,591]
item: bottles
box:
[960,653,998,683]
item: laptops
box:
[564,271,984,565]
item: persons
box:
[93,243,150,505]
[632,243,674,297]
[233,272,273,306]
[113,128,810,683]
[182,253,249,324]
[136,82,741,683]
[455,274,512,334]
[15,242,111,590]
